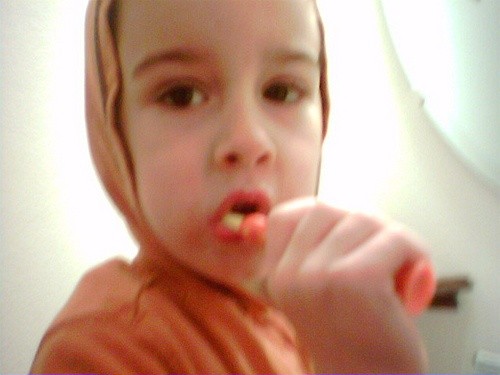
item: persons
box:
[26,0,433,375]
